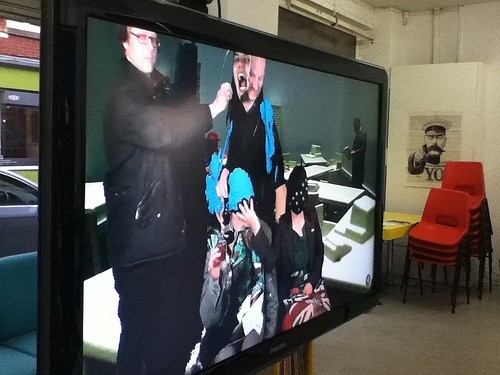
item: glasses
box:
[130,31,160,48]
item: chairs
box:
[400,159,494,315]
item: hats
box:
[422,120,451,135]
[287,165,309,215]
[226,167,253,212]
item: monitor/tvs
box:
[36,0,390,375]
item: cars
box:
[0,167,41,257]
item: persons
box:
[226,48,252,123]
[218,55,287,223]
[193,166,277,372]
[351,118,367,187]
[104,27,233,375]
[273,164,330,332]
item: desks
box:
[382,211,424,294]
[84,267,122,375]
[84,181,106,275]
[299,153,327,167]
[320,205,376,298]
[307,179,365,223]
[283,163,335,182]
